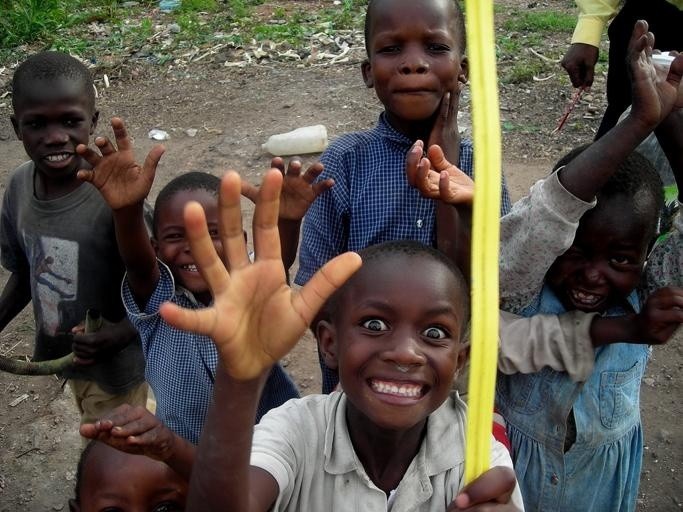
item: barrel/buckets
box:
[262,124,329,157]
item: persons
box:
[455,289,682,399]
[292,0,512,393]
[0,52,155,455]
[403,135,471,297]
[155,166,524,512]
[73,116,335,443]
[558,0,682,188]
[495,17,683,511]
[68,401,201,511]
